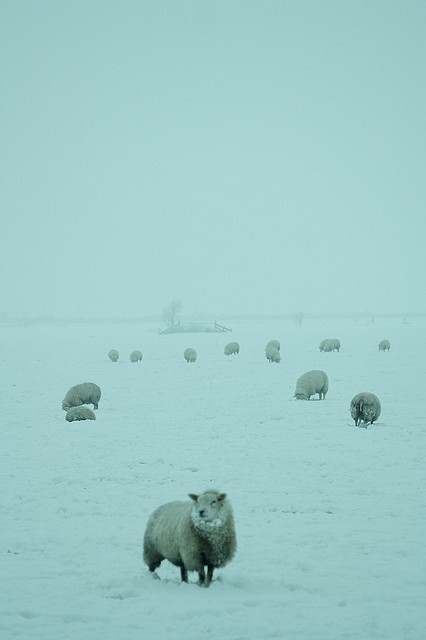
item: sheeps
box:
[143,489,237,589]
[66,406,95,422]
[378,339,391,352]
[130,351,142,363]
[184,348,197,363]
[319,339,329,352]
[266,347,281,363]
[265,340,279,352]
[224,342,241,357]
[108,350,119,362]
[349,392,381,427]
[323,339,341,352]
[293,370,329,402]
[62,382,101,411]
